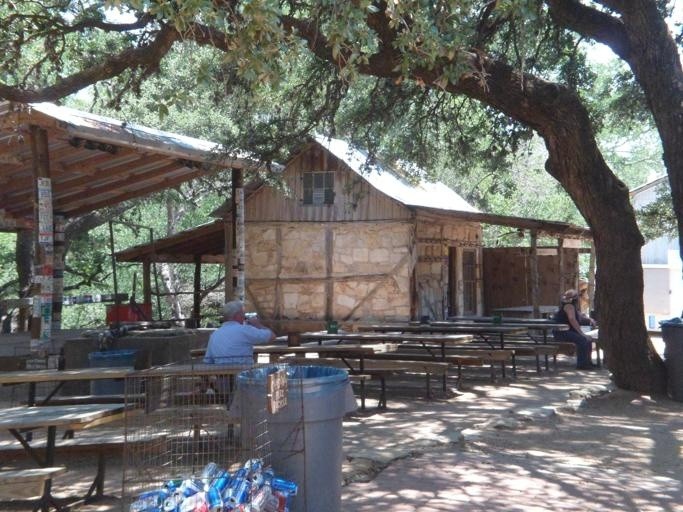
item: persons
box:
[553,290,597,371]
[199,300,276,395]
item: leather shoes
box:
[576,363,593,370]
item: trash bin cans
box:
[658,318,683,403]
[87,349,136,396]
[236,365,349,512]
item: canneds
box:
[130,458,299,512]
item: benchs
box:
[1,306,598,511]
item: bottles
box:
[648,313,656,329]
[130,496,161,512]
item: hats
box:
[561,289,578,303]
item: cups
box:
[287,331,300,347]
[325,322,338,334]
